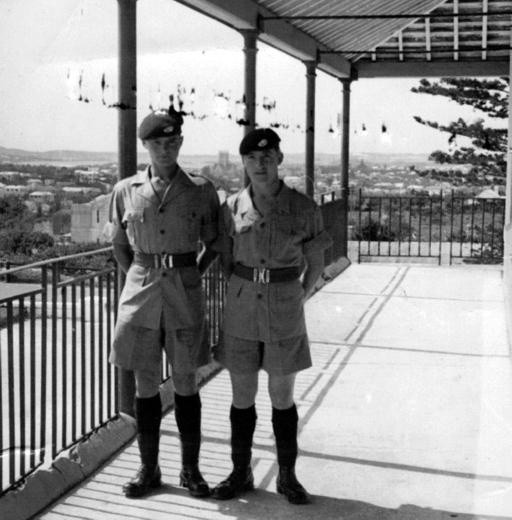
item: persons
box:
[99,108,225,500]
[209,126,333,506]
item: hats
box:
[240,128,281,155]
[138,108,181,139]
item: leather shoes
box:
[276,467,311,504]
[211,467,254,499]
[122,465,162,495]
[179,465,210,500]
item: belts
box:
[133,251,197,269]
[232,263,299,284]
